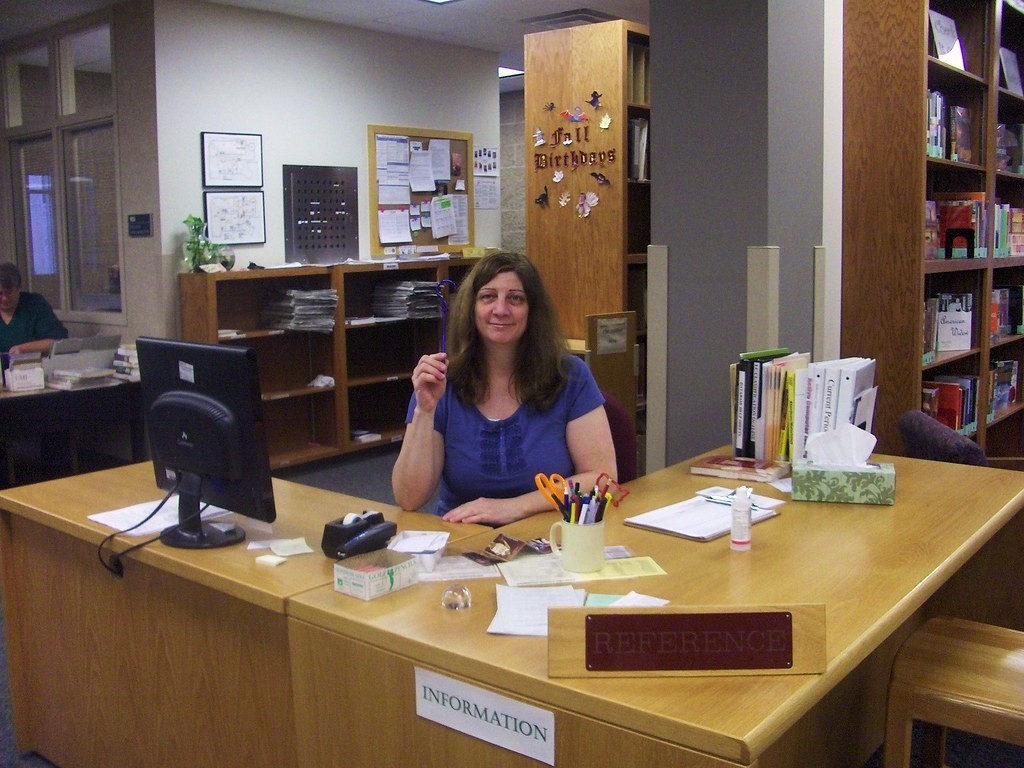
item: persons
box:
[0,263,68,481]
[391,251,618,528]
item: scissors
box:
[534,472,569,521]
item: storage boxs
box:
[462,247,498,257]
[333,549,420,601]
[387,530,451,574]
[3,366,45,391]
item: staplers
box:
[337,520,397,560]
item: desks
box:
[1,378,145,485]
[0,461,494,768]
[286,443,1023,768]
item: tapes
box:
[343,511,378,526]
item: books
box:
[730,348,877,465]
[930,10,967,70]
[46,345,140,391]
[690,455,792,480]
[628,119,649,180]
[370,279,444,319]
[1000,48,1024,96]
[997,124,1024,174]
[921,360,1018,436]
[924,293,973,365]
[261,288,339,332]
[989,285,1024,344]
[993,203,1024,258]
[926,89,973,164]
[925,191,989,259]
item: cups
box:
[549,516,606,573]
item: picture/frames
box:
[203,191,266,245]
[200,132,263,189]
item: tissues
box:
[791,422,896,506]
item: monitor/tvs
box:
[137,335,276,549]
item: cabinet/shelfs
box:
[523,19,651,435]
[179,257,482,472]
[841,0,1024,456]
[564,310,637,430]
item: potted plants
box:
[181,214,207,273]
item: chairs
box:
[899,409,987,468]
[600,392,637,485]
[885,615,1023,768]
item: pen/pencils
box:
[570,498,576,524]
[552,493,570,522]
[563,487,569,513]
[603,492,612,518]
[572,495,579,522]
[602,479,612,495]
[577,485,600,524]
[594,498,608,522]
[568,479,574,493]
[575,482,580,495]
[577,494,590,524]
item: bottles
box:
[730,488,752,552]
